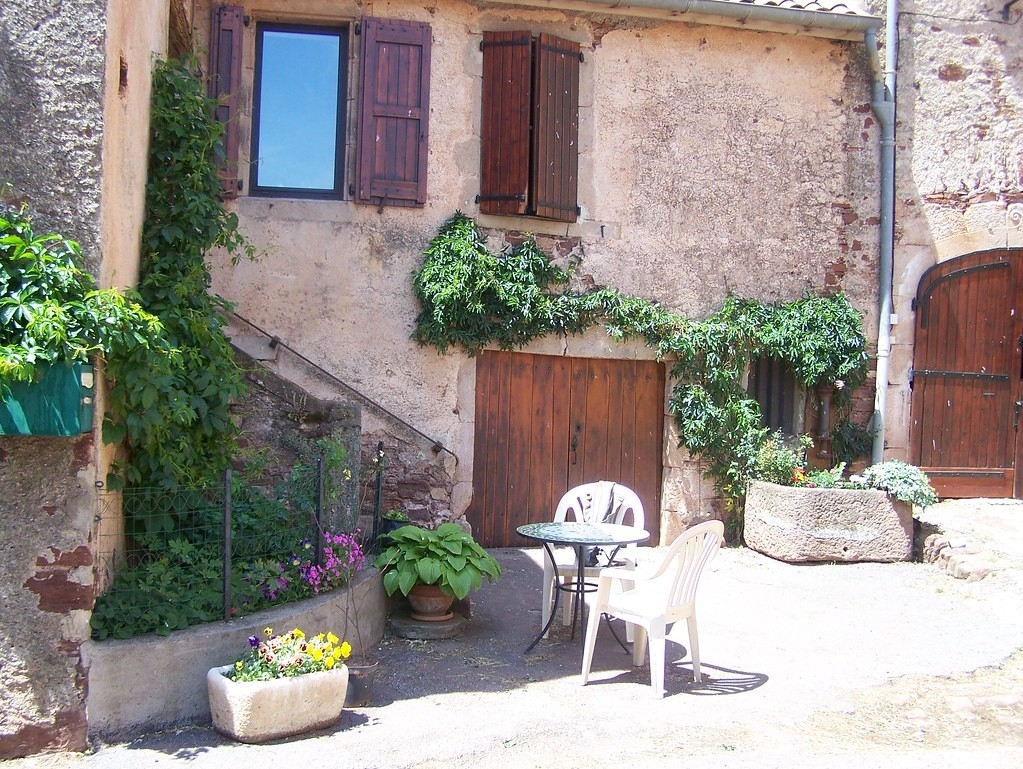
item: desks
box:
[515,521,650,657]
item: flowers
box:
[224,626,352,682]
[736,419,940,511]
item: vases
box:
[743,482,914,562]
[206,662,349,744]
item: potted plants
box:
[372,523,501,622]
[307,448,402,708]
[0,185,171,438]
[382,508,411,542]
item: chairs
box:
[540,483,645,642]
[581,520,725,700]
